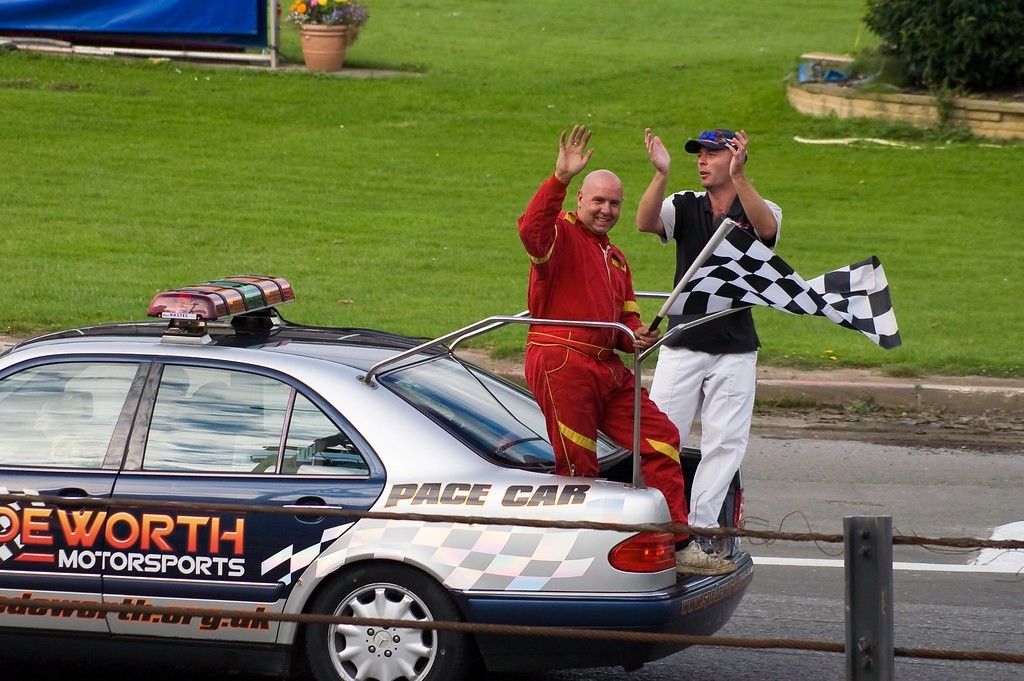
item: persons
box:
[518,126,738,573]
[636,128,783,558]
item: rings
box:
[574,142,579,146]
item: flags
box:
[665,226,902,350]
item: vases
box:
[300,24,348,72]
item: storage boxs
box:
[797,53,855,84]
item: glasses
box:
[699,130,721,140]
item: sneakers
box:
[690,535,717,556]
[675,539,737,575]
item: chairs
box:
[188,382,235,475]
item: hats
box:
[685,128,747,164]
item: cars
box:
[0,275,755,681]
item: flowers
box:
[284,0,370,48]
